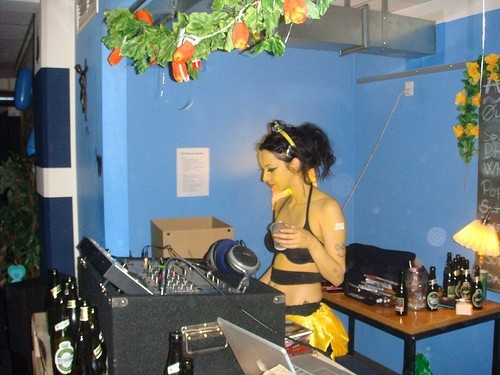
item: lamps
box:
[453,203,500,257]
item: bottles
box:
[426,265,439,312]
[163,331,187,375]
[46,268,108,375]
[395,271,409,316]
[184,357,195,375]
[442,253,484,310]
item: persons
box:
[256,120,349,361]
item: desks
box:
[317,280,500,375]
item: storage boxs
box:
[151,216,233,261]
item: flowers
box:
[102,0,330,82]
[452,52,500,162]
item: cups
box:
[269,221,289,250]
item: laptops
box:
[217,318,357,375]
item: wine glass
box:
[411,281,425,309]
